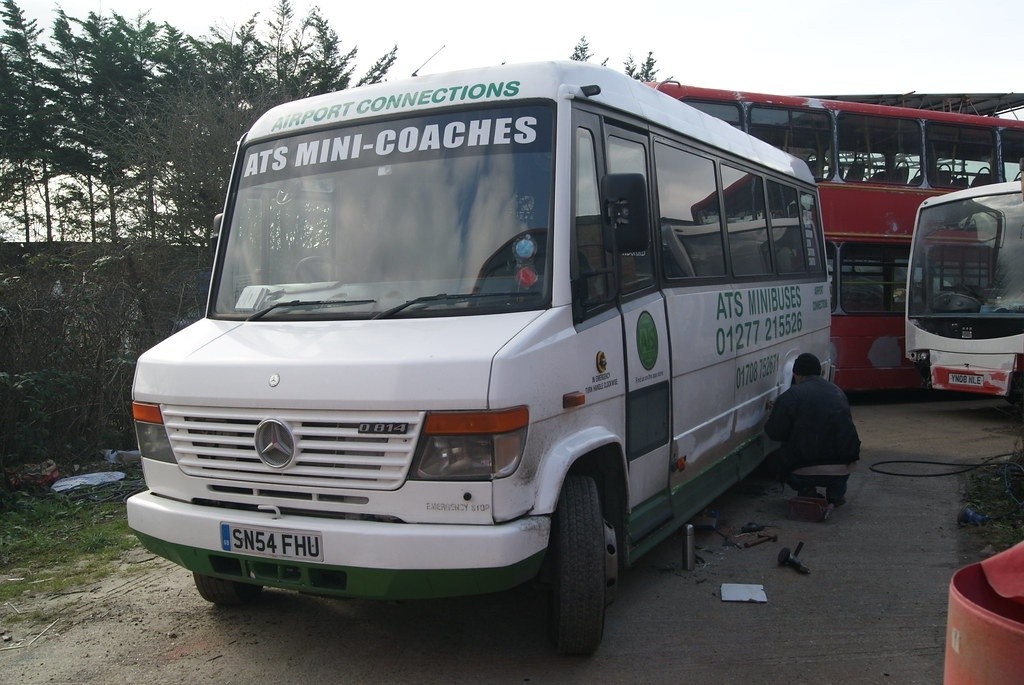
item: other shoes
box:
[798,488,825,499]
[828,498,847,506]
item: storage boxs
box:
[786,496,828,523]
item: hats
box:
[793,353,822,375]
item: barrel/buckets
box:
[943,562,1024,685]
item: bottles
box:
[996,297,1001,307]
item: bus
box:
[901,180,1024,413]
[126,55,830,665]
[660,219,822,279]
[641,80,1024,403]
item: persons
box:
[764,353,861,508]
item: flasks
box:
[682,524,695,570]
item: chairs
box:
[803,153,1024,188]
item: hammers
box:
[777,541,810,574]
[744,533,778,548]
